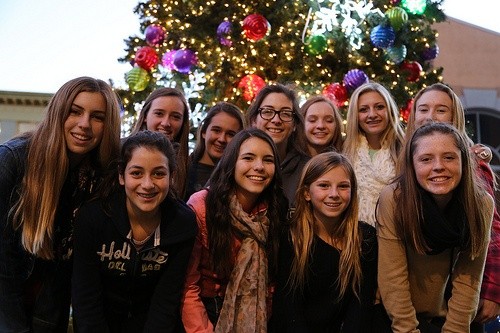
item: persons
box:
[181,126,282,333]
[245,84,310,209]
[120,87,189,199]
[341,82,492,227]
[185,102,245,203]
[295,96,345,156]
[403,84,500,333]
[375,120,494,333]
[0,75,121,333]
[68,132,198,333]
[267,152,378,333]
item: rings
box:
[480,151,488,158]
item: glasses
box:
[257,108,295,122]
